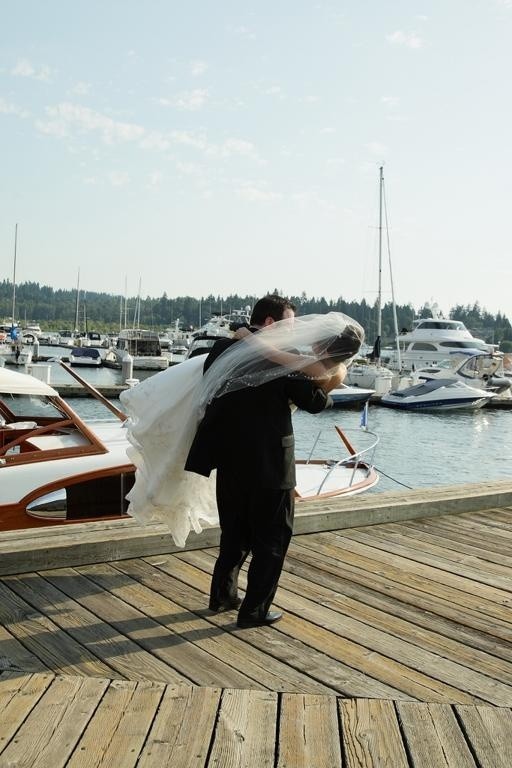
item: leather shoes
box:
[211,592,244,612]
[239,612,283,629]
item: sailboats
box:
[0,164,512,414]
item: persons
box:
[120,312,366,550]
[184,296,334,628]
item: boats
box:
[0,352,384,533]
